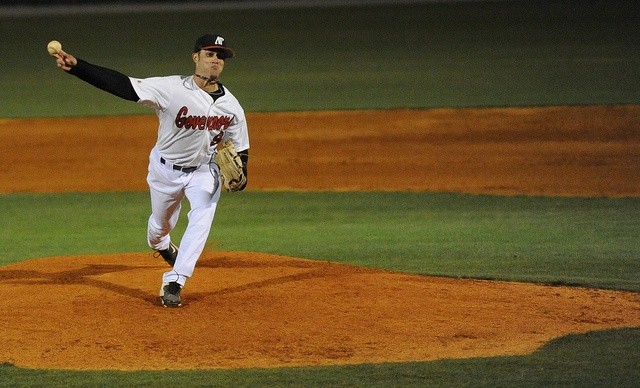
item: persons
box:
[49,35,250,309]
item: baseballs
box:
[47,40,61,54]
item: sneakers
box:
[153,242,178,266]
[160,281,182,307]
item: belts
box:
[161,156,198,172]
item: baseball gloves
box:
[209,139,246,193]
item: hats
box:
[195,34,233,57]
